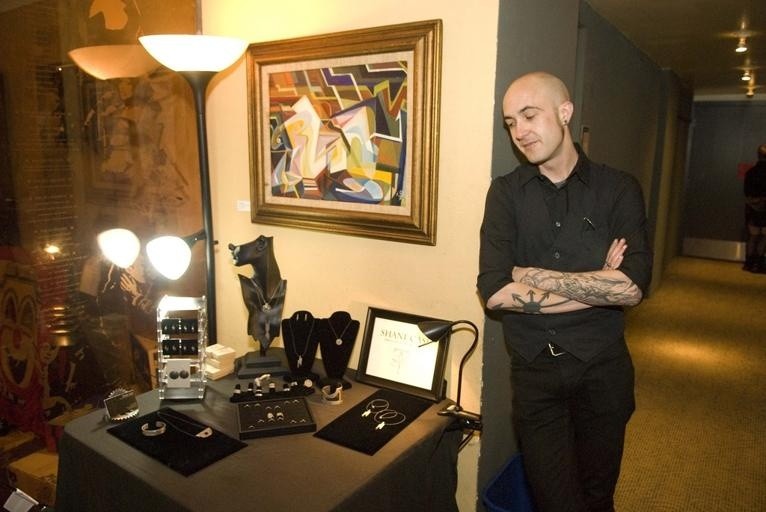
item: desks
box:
[63,347,462,512]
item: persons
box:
[476,71,657,512]
[227,235,287,352]
[117,273,178,326]
[744,143,766,275]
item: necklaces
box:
[252,281,284,314]
[326,316,351,345]
[250,275,282,312]
[286,317,315,368]
[156,411,211,439]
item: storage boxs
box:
[8,452,59,506]
[0,429,41,469]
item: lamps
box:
[67,44,160,271]
[416,315,481,448]
[138,32,250,346]
[716,13,766,97]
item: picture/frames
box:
[354,306,453,402]
[244,19,444,247]
[75,66,194,215]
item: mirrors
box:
[0,2,220,471]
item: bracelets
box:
[140,419,166,436]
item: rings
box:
[244,398,307,430]
[232,382,291,398]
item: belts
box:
[539,342,568,359]
